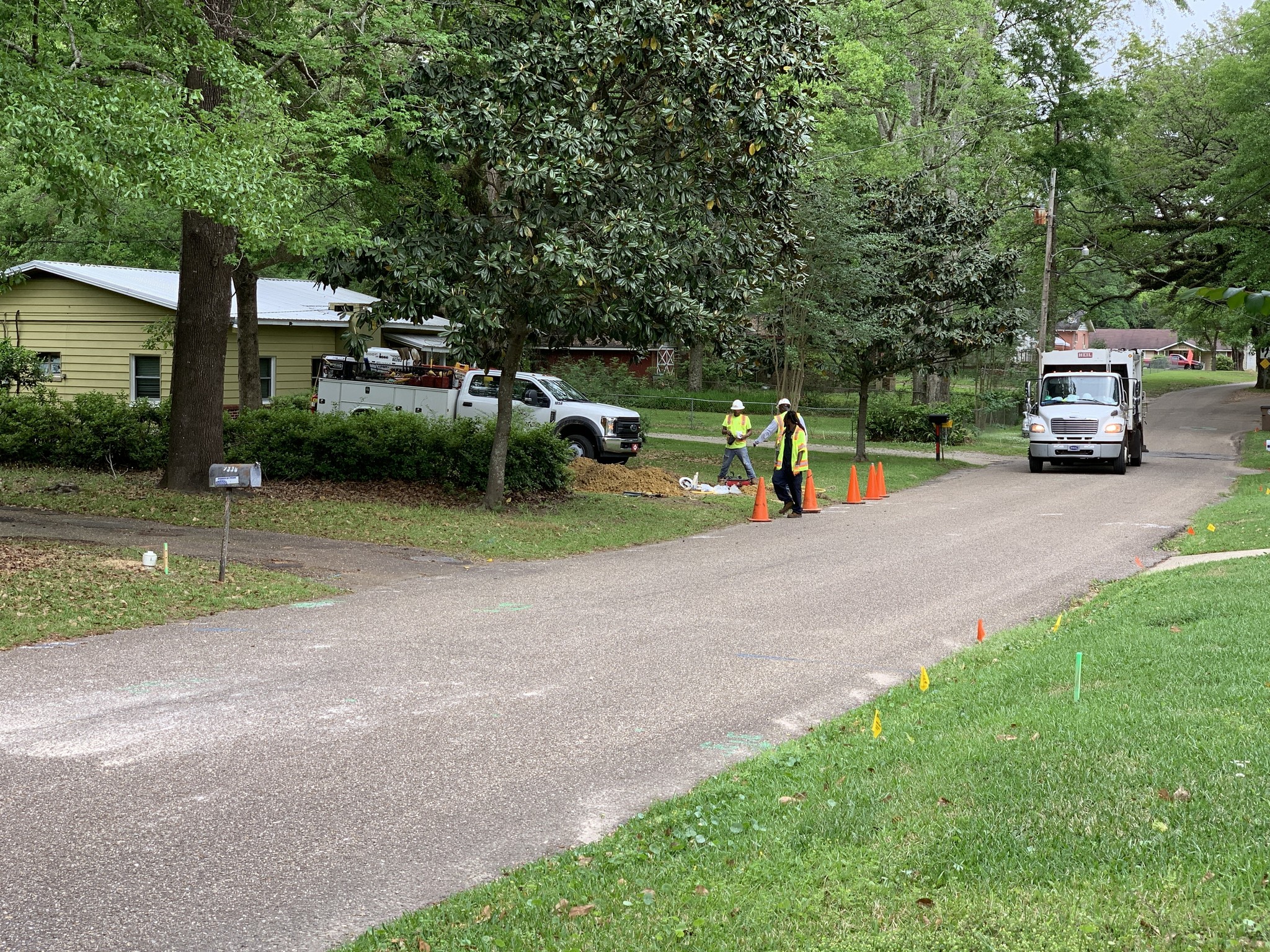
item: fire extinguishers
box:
[311,389,318,414]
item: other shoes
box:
[751,477,757,484]
[717,476,723,482]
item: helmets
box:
[776,398,791,410]
[731,400,745,410]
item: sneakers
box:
[779,501,794,514]
[787,511,802,518]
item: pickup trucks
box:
[308,353,646,468]
[1144,354,1203,370]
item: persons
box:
[772,411,809,519]
[717,399,759,486]
[753,398,808,494]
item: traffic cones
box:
[876,461,890,497]
[841,464,866,504]
[801,468,823,513]
[747,476,773,521]
[861,462,883,500]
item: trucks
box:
[1021,346,1148,475]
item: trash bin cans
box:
[1261,405,1270,431]
